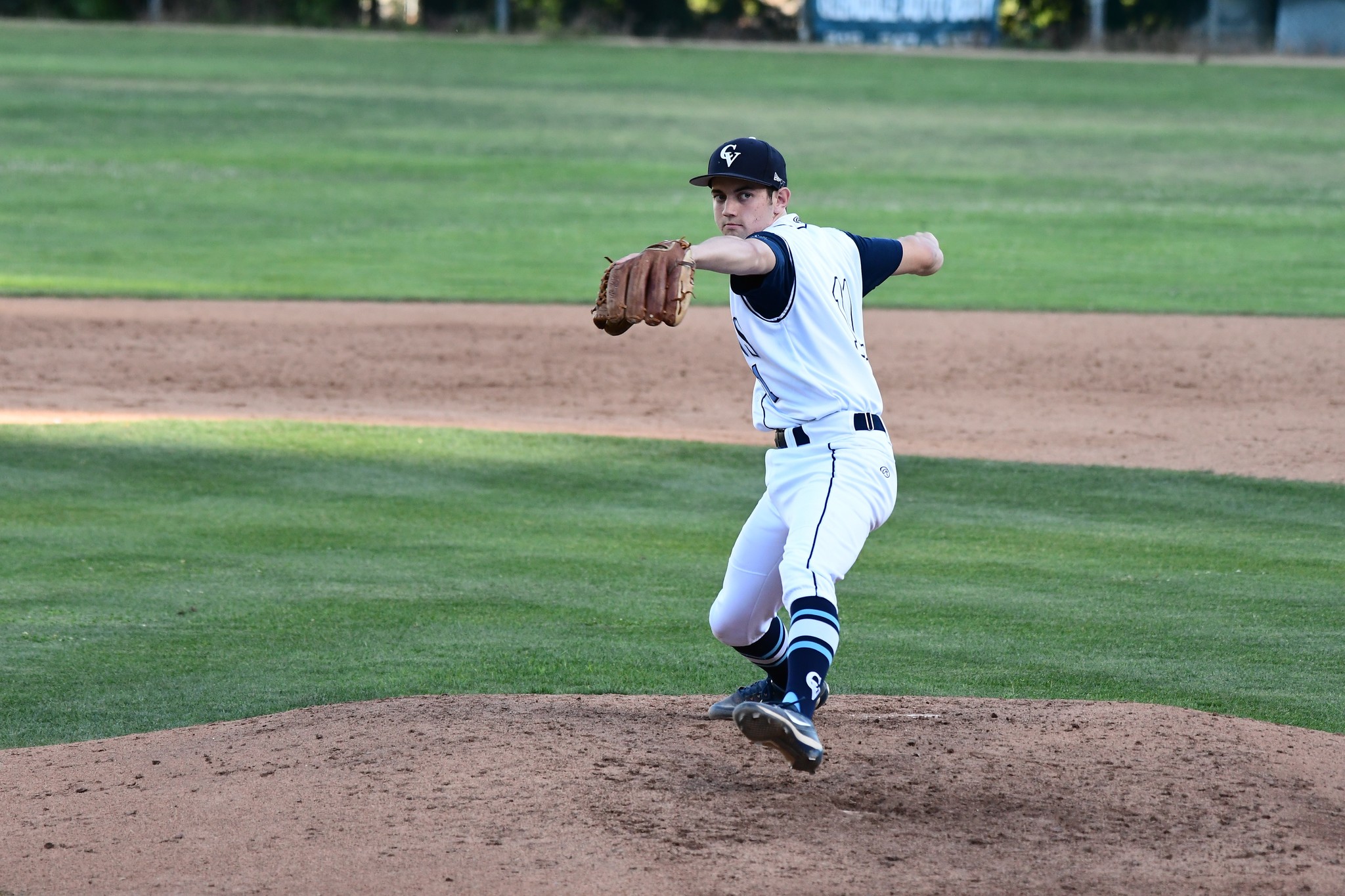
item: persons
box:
[591,138,946,775]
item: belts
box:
[774,413,884,448]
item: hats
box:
[689,137,788,190]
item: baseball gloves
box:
[590,236,696,335]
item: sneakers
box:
[732,702,820,775]
[708,678,829,720]
